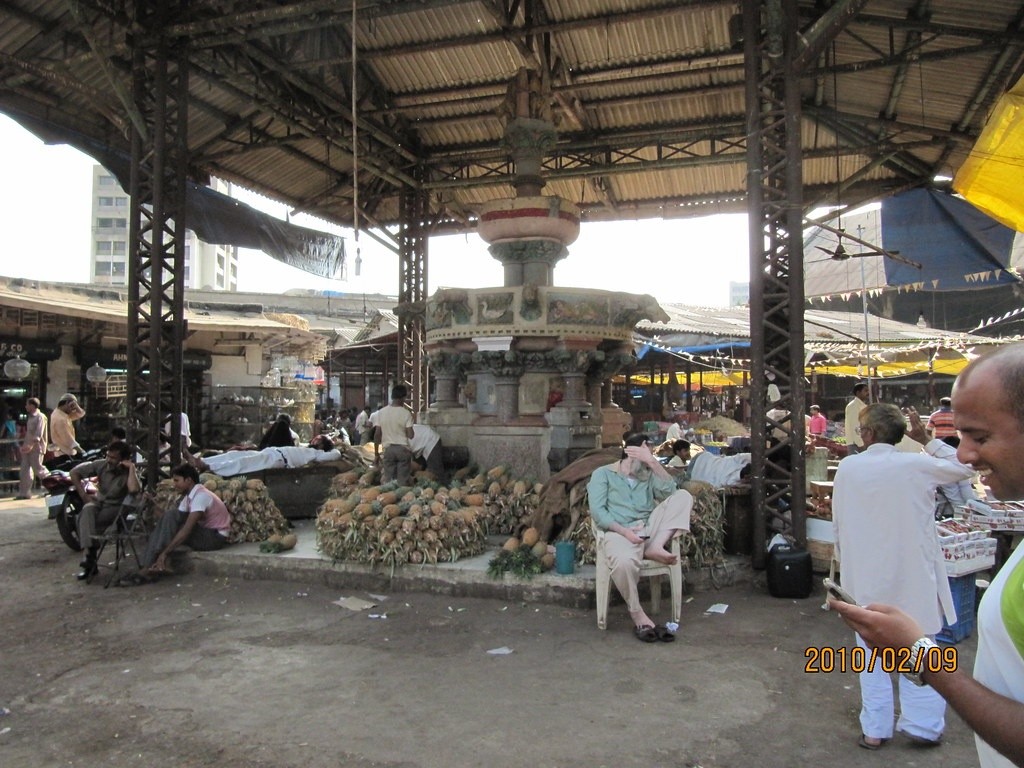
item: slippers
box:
[859,733,888,750]
[899,730,942,745]
[632,625,675,642]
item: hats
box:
[59,393,77,400]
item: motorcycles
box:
[42,443,110,552]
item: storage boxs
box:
[935,573,975,644]
[705,445,729,454]
[935,498,1024,577]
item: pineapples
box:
[316,466,543,565]
[157,472,298,555]
[680,479,713,497]
[487,527,554,580]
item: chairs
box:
[86,492,148,589]
[590,518,682,629]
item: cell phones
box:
[639,536,650,539]
[824,577,864,609]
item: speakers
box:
[767,547,812,599]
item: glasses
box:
[855,426,875,435]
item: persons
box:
[589,436,693,631]
[200,435,341,476]
[71,442,141,580]
[845,383,869,455]
[97,428,126,460]
[810,405,826,439]
[314,406,383,446]
[0,410,17,439]
[50,393,85,457]
[686,452,751,488]
[926,397,976,521]
[257,414,300,451]
[833,404,978,748]
[726,403,742,423]
[407,424,444,473]
[374,385,415,486]
[666,416,702,446]
[668,439,691,471]
[165,400,192,448]
[120,463,230,586]
[830,342,1023,768]
[14,398,50,499]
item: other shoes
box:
[77,554,98,579]
[13,496,30,500]
[120,571,159,586]
[150,560,185,574]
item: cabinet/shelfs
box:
[208,385,315,451]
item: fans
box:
[809,40,900,261]
